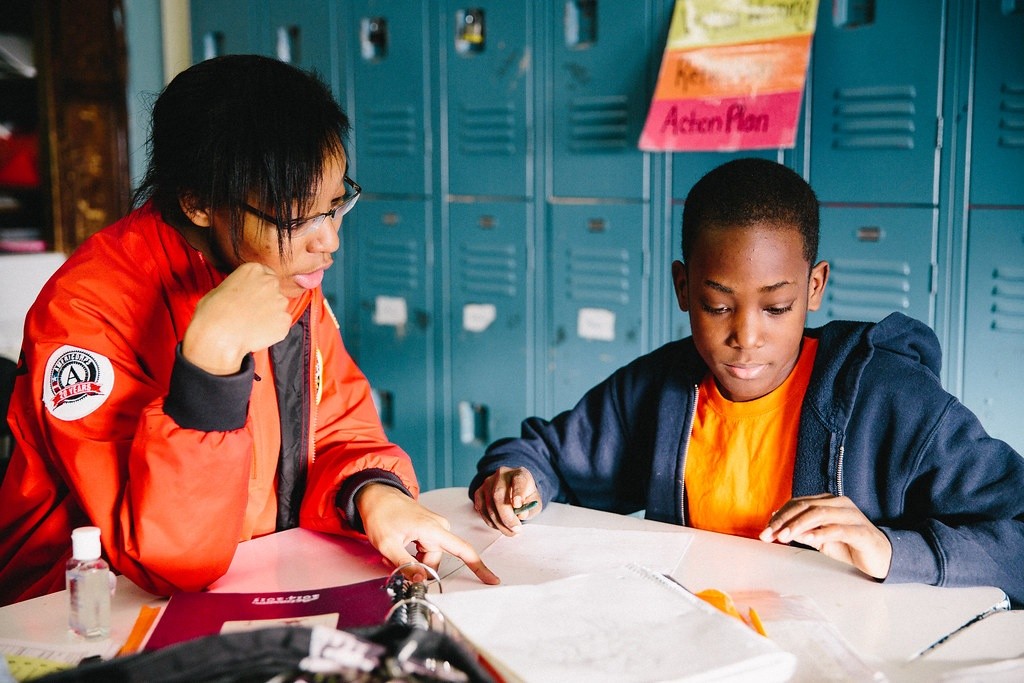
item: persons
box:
[467,156,1024,601]
[0,54,500,605]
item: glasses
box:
[234,174,361,239]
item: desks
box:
[0,490,1011,683]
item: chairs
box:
[187,0,1024,474]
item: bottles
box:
[65,526,110,639]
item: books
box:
[422,562,799,683]
[115,575,426,657]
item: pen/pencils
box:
[515,500,538,515]
